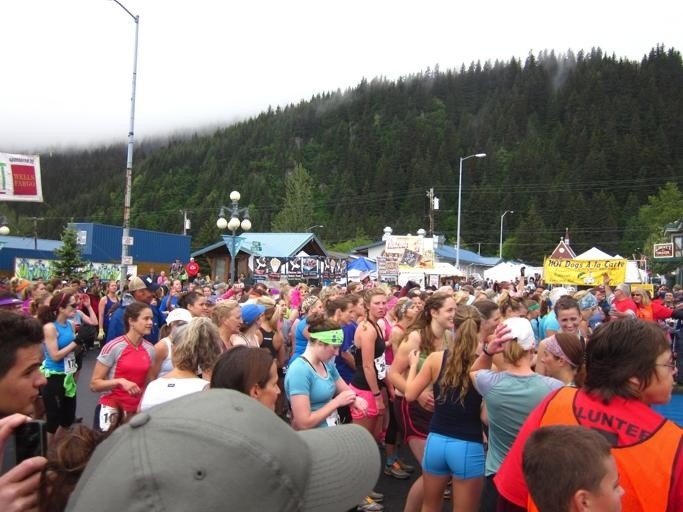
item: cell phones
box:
[15,419,46,466]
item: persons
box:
[0,256,683,511]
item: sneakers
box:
[384,457,414,479]
[358,492,385,512]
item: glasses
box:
[632,294,640,296]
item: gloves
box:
[73,324,97,346]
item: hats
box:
[496,317,535,352]
[166,308,191,326]
[360,272,370,280]
[128,276,158,291]
[242,304,266,324]
[65,387,382,512]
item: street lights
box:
[455,153,487,270]
[306,224,324,233]
[500,209,514,260]
[216,190,252,283]
[0,216,10,235]
[111,0,140,296]
